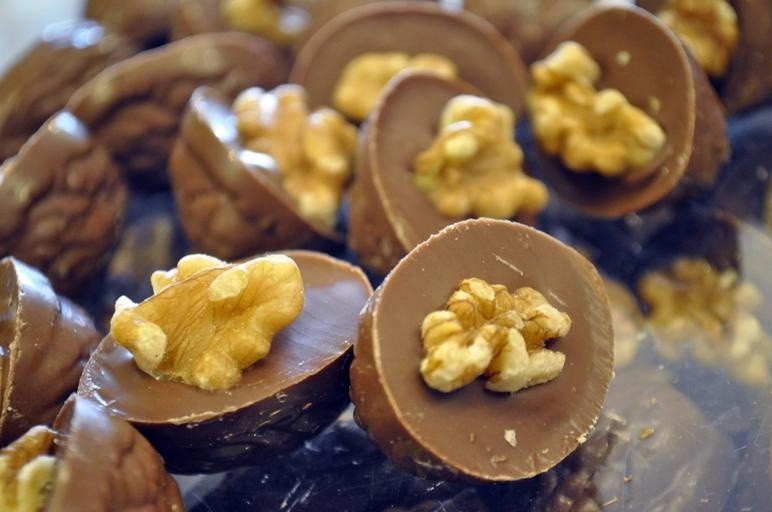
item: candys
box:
[0,0,772,512]
